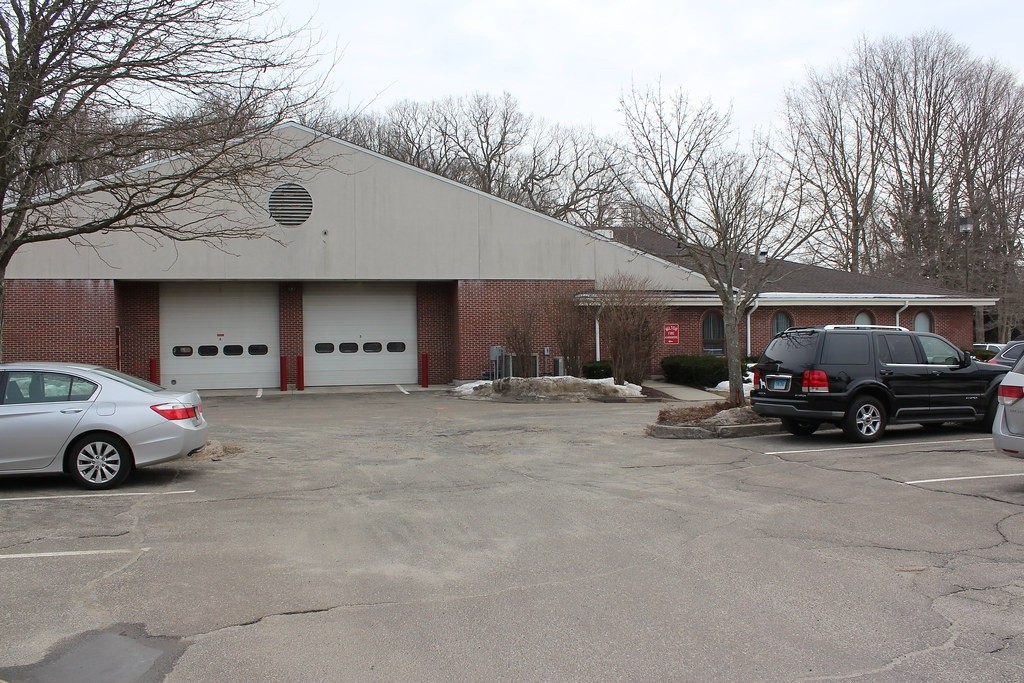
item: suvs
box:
[749,324,1012,443]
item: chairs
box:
[5,379,24,402]
[27,377,43,399]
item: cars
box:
[0,360,209,489]
[973,341,1024,458]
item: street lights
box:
[959,217,973,291]
